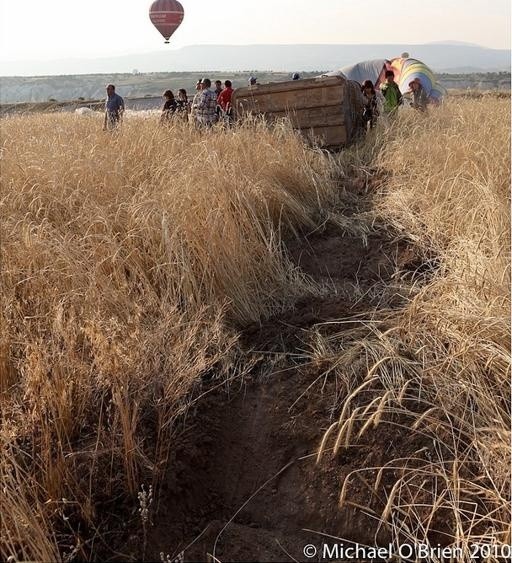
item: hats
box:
[409,77,422,87]
[248,76,257,82]
[293,73,299,80]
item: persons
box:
[361,79,386,131]
[101,84,124,131]
[379,70,403,116]
[408,77,427,112]
[159,76,262,132]
